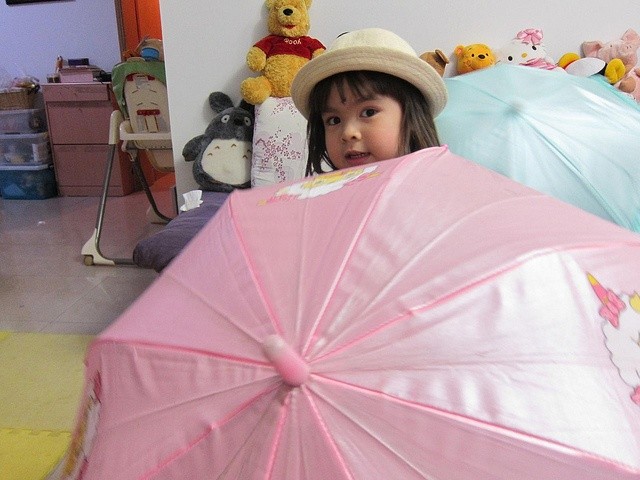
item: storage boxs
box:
[53,145,135,197]
[0,161,56,200]
[0,107,47,134]
[0,132,52,166]
[40,82,119,144]
[0,84,40,110]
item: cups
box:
[32,142,50,161]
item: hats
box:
[290,27,449,121]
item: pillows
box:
[251,97,334,189]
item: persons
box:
[303,27,440,177]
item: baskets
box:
[0,92,33,110]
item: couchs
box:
[133,86,334,271]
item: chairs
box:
[81,59,177,266]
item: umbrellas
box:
[433,61,639,232]
[63,143,639,480]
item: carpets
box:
[1,332,95,479]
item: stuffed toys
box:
[418,49,449,78]
[240,0,326,105]
[495,28,566,74]
[181,91,255,193]
[454,43,495,74]
[558,52,626,86]
[581,27,639,104]
